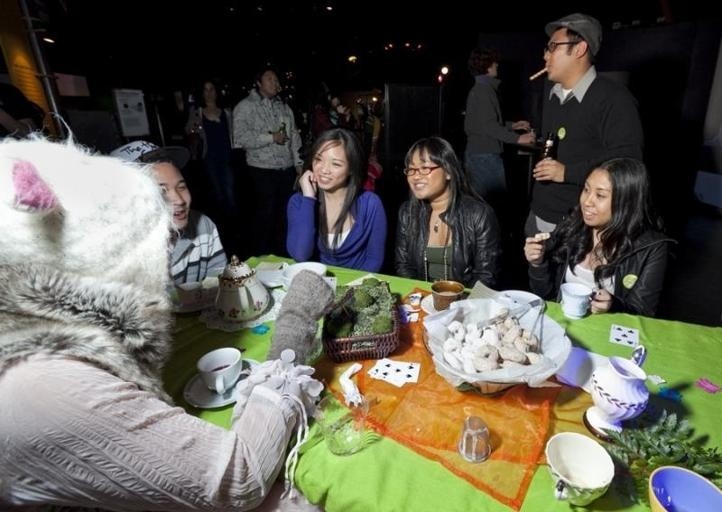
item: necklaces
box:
[426,211,446,234]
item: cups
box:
[647,465,721,512]
[528,128,538,134]
[459,414,491,462]
[314,392,366,457]
[559,284,592,319]
[195,346,242,395]
[177,281,202,306]
[544,431,615,508]
[429,279,465,310]
[200,275,219,303]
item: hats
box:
[545,13,603,56]
[110,138,191,170]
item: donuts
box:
[442,308,544,374]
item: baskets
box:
[318,286,402,363]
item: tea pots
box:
[215,254,271,324]
[582,344,651,443]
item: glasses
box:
[403,165,440,176]
[545,41,576,52]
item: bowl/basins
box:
[281,260,327,286]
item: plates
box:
[173,301,214,313]
[499,289,544,314]
[419,289,449,317]
[183,358,260,410]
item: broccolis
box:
[322,276,391,338]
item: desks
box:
[155,254,722,512]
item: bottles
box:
[538,132,555,185]
[277,122,286,145]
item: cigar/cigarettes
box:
[529,65,551,82]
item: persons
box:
[1,133,334,511]
[284,127,387,273]
[521,13,645,249]
[462,44,536,200]
[110,139,228,289]
[1,81,45,140]
[393,134,499,291]
[232,65,306,264]
[523,154,680,318]
[188,77,235,206]
